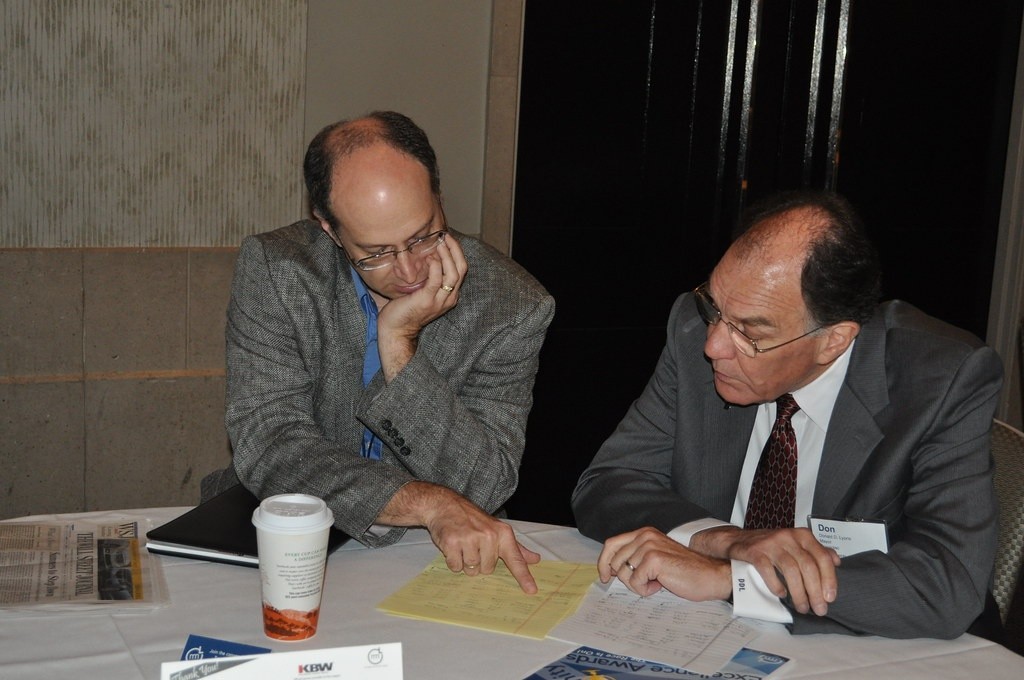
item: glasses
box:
[693,282,824,357]
[333,195,448,270]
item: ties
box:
[743,394,801,530]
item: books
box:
[145,482,352,570]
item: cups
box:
[252,494,334,642]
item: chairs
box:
[992,419,1024,641]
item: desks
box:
[0,506,1024,680]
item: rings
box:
[441,284,454,293]
[463,563,481,569]
[625,560,634,571]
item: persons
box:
[200,110,556,596]
[569,192,1004,641]
